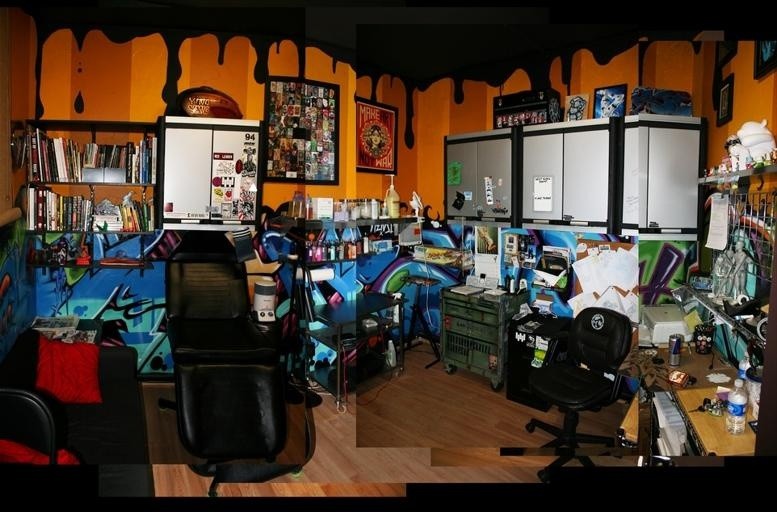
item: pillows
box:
[37,334,103,404]
[0,435,89,471]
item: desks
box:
[35,318,104,345]
[637,337,760,469]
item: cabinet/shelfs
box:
[160,116,261,226]
[442,127,518,226]
[520,117,621,235]
[441,280,532,391]
[274,192,425,266]
[684,159,777,348]
[505,311,574,415]
[308,294,409,407]
[25,121,158,273]
[623,115,709,242]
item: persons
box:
[367,126,384,150]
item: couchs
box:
[1,326,154,509]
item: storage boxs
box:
[493,88,563,128]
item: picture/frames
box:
[265,77,340,186]
[593,84,626,120]
[714,39,739,72]
[754,40,777,78]
[716,72,735,126]
[356,98,400,176]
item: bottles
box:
[340,197,380,221]
[385,339,397,368]
[738,352,751,378]
[305,237,368,264]
[304,196,313,221]
[287,196,297,218]
[724,379,748,435]
[296,196,305,217]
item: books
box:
[18,124,157,236]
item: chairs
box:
[160,230,322,500]
[523,301,632,479]
